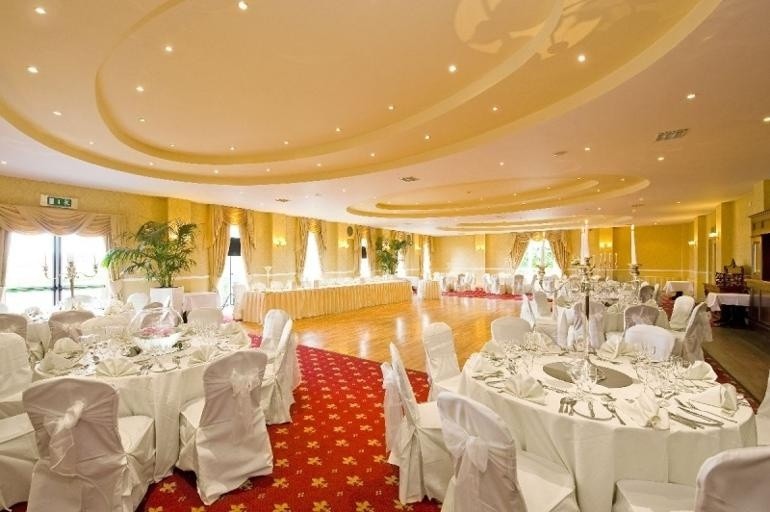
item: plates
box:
[151,363,177,372]
[579,380,611,396]
[573,401,613,421]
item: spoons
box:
[608,402,626,426]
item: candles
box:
[582,221,589,258]
[630,225,636,264]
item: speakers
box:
[362,246,366,258]
[228,238,241,256]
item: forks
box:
[687,401,738,424]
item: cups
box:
[721,383,737,411]
[108,338,120,359]
[109,279,125,308]
[654,408,670,432]
[79,336,91,363]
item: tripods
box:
[218,256,236,312]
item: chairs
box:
[259,308,299,420]
[525,291,709,364]
[422,322,459,403]
[381,340,464,502]
[488,315,535,353]
[436,391,576,512]
[126,293,151,309]
[16,379,157,512]
[0,301,227,349]
[618,444,766,511]
[0,331,41,512]
[179,350,275,502]
[433,269,538,298]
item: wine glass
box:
[525,333,542,379]
[585,366,598,400]
[498,336,515,370]
[675,360,693,392]
[656,361,674,400]
[632,342,644,366]
[642,345,656,372]
[634,364,652,397]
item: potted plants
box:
[372,237,409,277]
[98,216,202,289]
